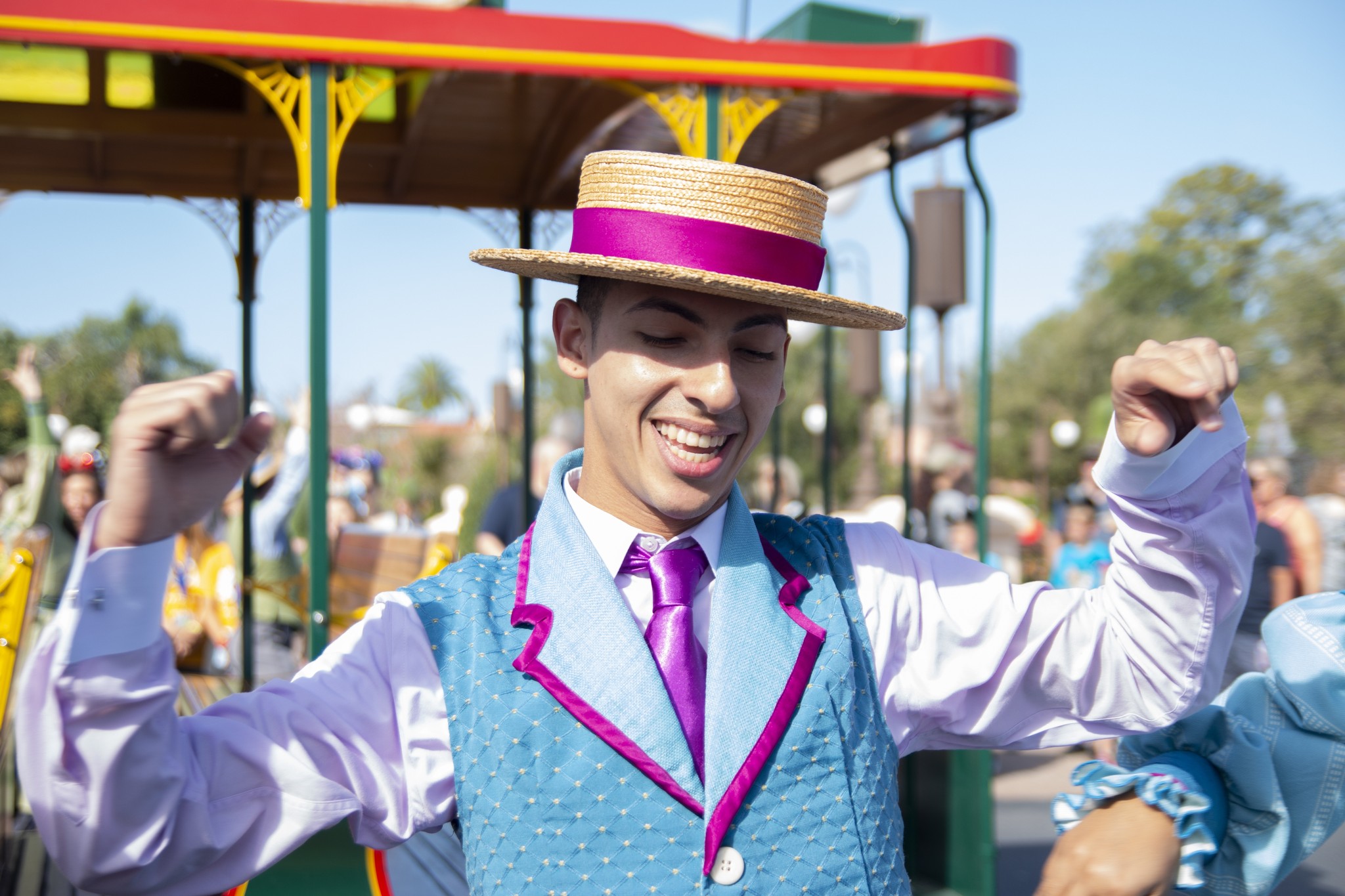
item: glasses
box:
[1248,478,1270,490]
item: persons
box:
[946,515,998,568]
[160,396,381,681]
[12,148,1260,896]
[1034,591,1344,896]
[474,436,574,557]
[1044,455,1119,589]
[1217,456,1345,698]
[0,454,103,714]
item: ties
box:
[619,540,714,785]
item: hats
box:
[471,148,907,332]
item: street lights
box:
[912,178,968,414]
[803,403,828,501]
[1051,417,1084,501]
